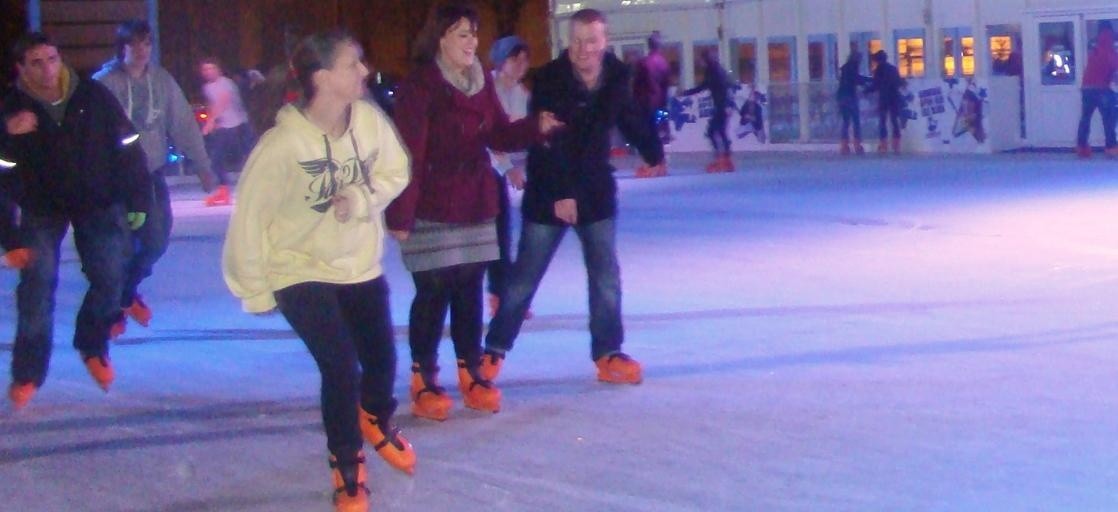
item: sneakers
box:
[488,294,534,321]
[705,154,735,173]
[326,450,372,512]
[357,401,419,472]
[77,348,115,384]
[636,159,671,179]
[204,183,231,205]
[109,293,152,338]
[9,380,37,402]
[595,351,643,375]
[1075,143,1116,160]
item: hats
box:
[487,34,522,65]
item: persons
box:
[1075,27,1118,158]
[836,51,870,155]
[735,83,768,144]
[218,1,740,509]
[91,18,219,336]
[0,33,149,406]
[859,48,904,157]
[194,53,257,205]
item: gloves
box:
[3,247,33,271]
[127,212,146,231]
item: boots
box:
[878,136,901,155]
[409,360,453,412]
[455,353,504,405]
[838,134,865,156]
